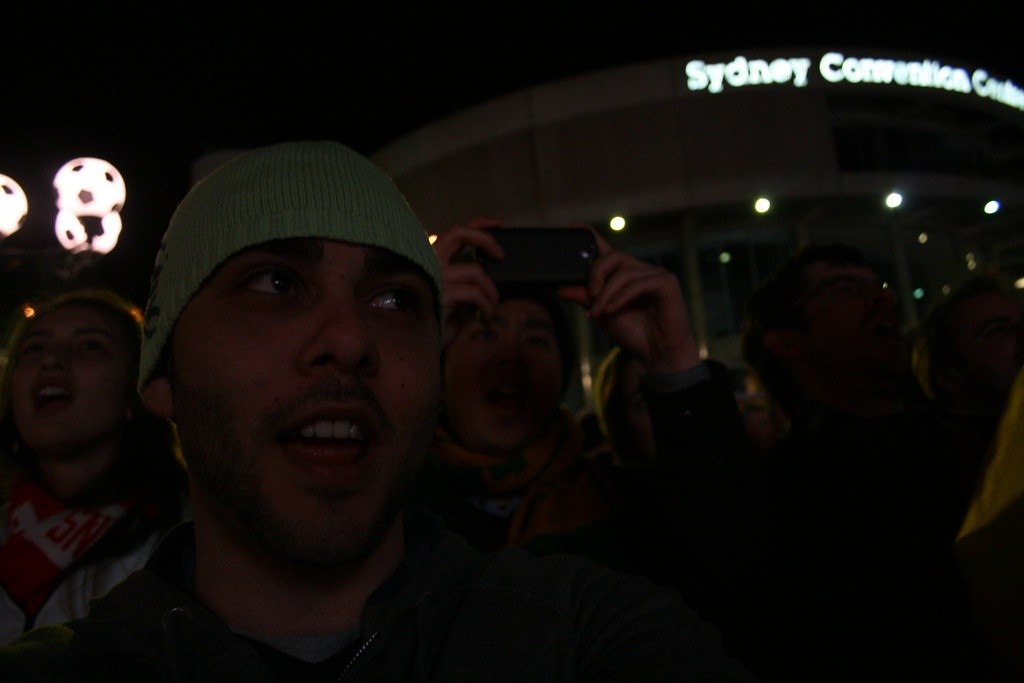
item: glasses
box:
[783,277,903,318]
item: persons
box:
[1,140,759,683]
[0,292,204,648]
[414,214,1024,683]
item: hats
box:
[138,140,443,427]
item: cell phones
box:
[475,225,598,293]
